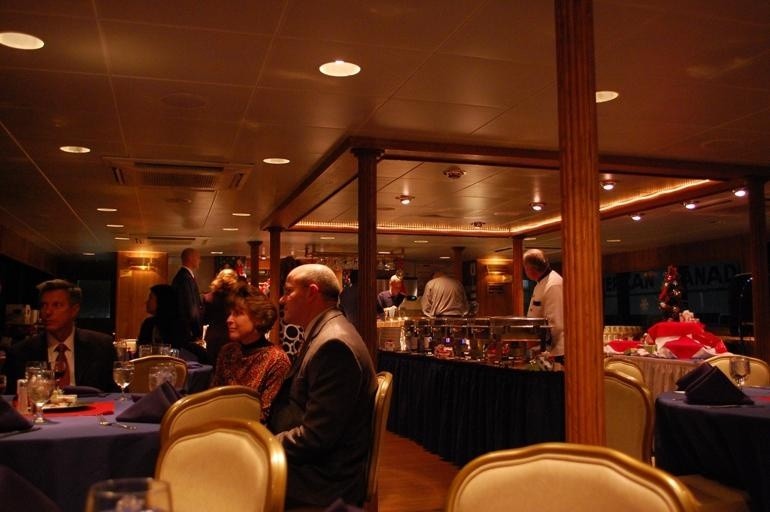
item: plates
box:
[39,402,91,411]
[379,325,407,352]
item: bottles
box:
[410,322,542,365]
[16,379,28,414]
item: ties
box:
[53,343,71,390]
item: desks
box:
[377,347,565,469]
[185,360,212,394]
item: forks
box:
[96,411,137,429]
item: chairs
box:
[600,335,770,461]
[161,386,263,446]
[445,440,706,512]
[363,371,393,511]
[124,355,188,392]
[146,417,287,511]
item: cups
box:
[603,325,643,343]
[137,343,179,390]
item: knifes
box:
[707,402,763,409]
[0,426,42,438]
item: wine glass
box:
[25,360,66,424]
[113,360,136,402]
[728,356,751,393]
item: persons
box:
[420,268,472,317]
[171,246,203,339]
[134,282,204,361]
[377,274,408,313]
[199,268,244,365]
[260,263,380,511]
[339,269,385,324]
[4,278,122,393]
[206,280,293,424]
[520,247,564,366]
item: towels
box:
[64,385,101,396]
[0,396,35,433]
